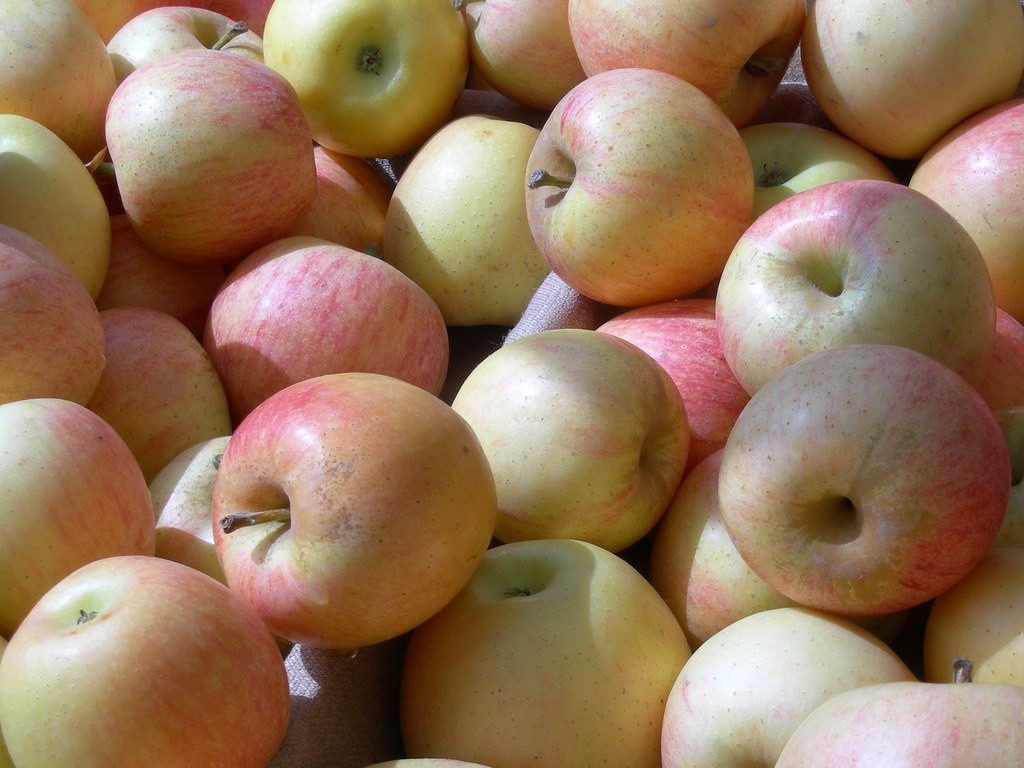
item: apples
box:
[0,0,1024,768]
